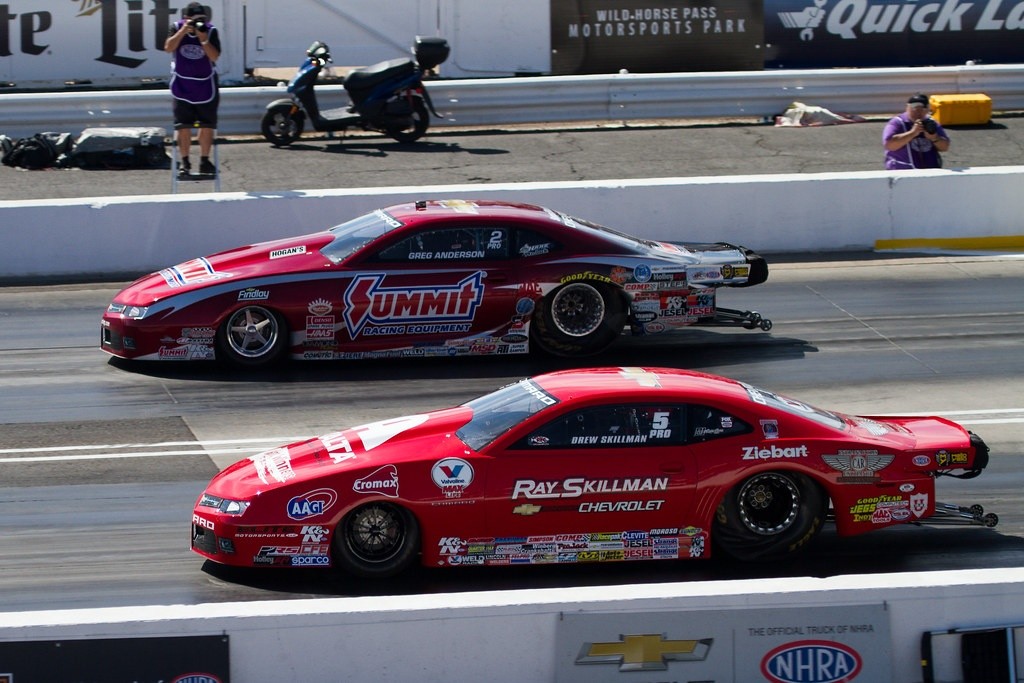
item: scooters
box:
[260,41,451,144]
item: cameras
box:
[921,119,938,135]
[189,18,208,32]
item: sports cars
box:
[99,198,773,374]
[190,366,1001,594]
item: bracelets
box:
[932,140,938,143]
[201,40,208,45]
[930,138,937,141]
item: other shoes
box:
[178,164,189,174]
[199,162,219,173]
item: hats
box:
[908,94,928,108]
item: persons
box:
[882,94,950,170]
[165,2,222,175]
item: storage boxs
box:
[928,93,993,126]
[411,33,452,69]
